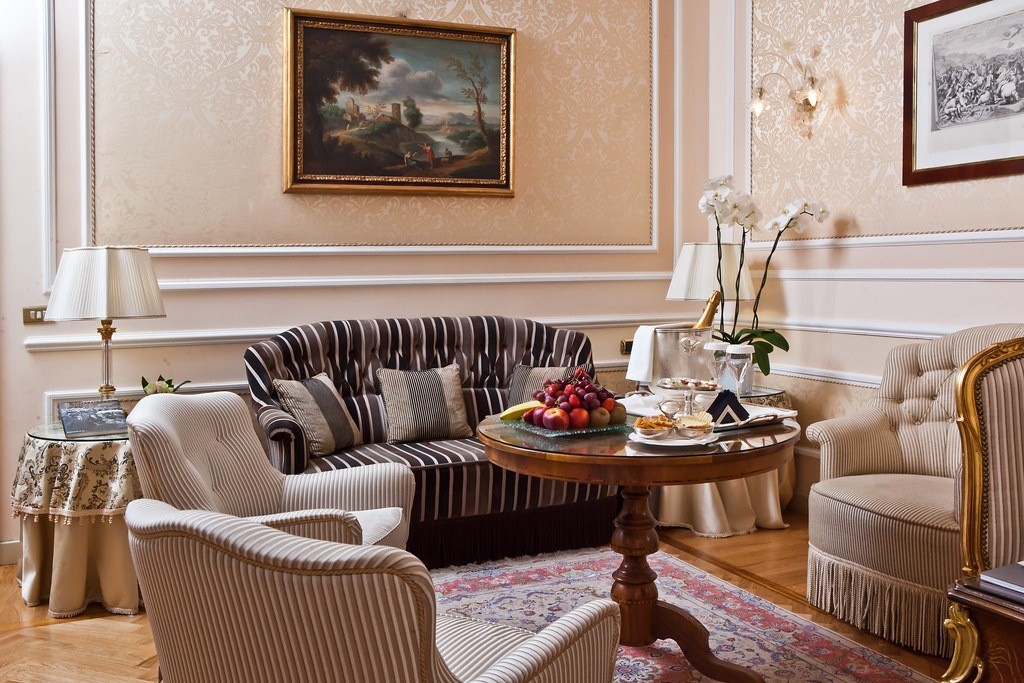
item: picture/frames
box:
[282,8,516,198]
[902,0,1024,187]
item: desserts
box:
[663,377,718,388]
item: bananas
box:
[499,400,546,420]
[500,436,524,446]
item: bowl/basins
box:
[632,426,674,439]
[674,426,713,439]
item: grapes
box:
[531,368,607,411]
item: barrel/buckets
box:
[648,329,715,394]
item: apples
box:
[533,407,589,430]
[565,441,627,455]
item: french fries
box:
[634,417,672,430]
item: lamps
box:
[43,245,169,402]
[663,241,757,301]
[751,75,821,118]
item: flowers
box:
[141,376,191,395]
[696,170,829,344]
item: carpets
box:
[422,545,939,683]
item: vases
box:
[704,352,756,397]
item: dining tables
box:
[476,400,803,683]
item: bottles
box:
[693,291,721,328]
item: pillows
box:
[507,365,591,409]
[375,363,475,445]
[274,372,365,456]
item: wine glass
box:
[703,342,753,405]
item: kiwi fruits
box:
[590,402,627,427]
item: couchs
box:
[125,497,622,683]
[806,325,1024,659]
[125,391,416,550]
[244,315,629,569]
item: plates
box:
[629,432,719,447]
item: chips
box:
[674,411,713,429]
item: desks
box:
[651,388,802,535]
[9,427,148,617]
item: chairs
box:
[940,337,1024,683]
